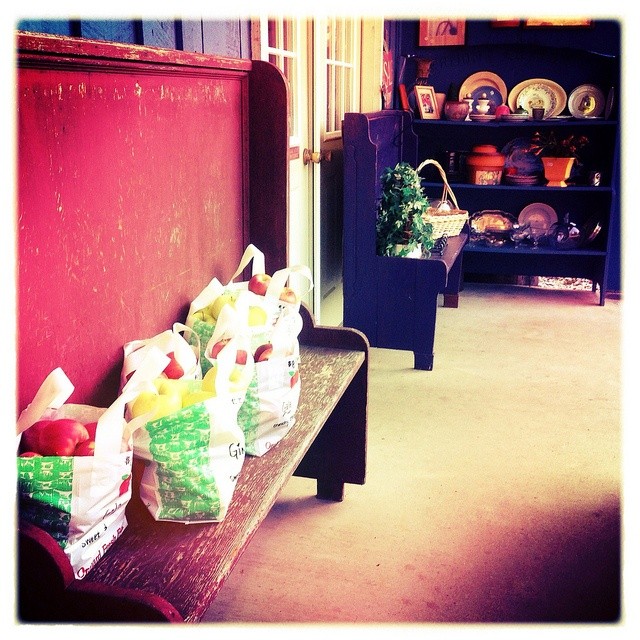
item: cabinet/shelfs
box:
[381,17,616,307]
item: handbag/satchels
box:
[17,346,171,580]
[118,322,203,398]
[182,243,313,379]
[123,332,255,524]
[205,291,303,457]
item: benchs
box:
[18,30,373,622]
[341,109,460,372]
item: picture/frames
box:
[418,17,465,47]
[490,17,521,27]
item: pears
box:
[132,369,240,422]
[182,296,287,332]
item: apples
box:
[121,335,308,397]
[278,284,301,305]
[226,266,274,298]
[18,415,107,459]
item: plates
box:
[470,85,503,115]
[568,84,605,119]
[459,71,507,115]
[507,77,567,122]
[516,83,557,119]
[604,86,615,119]
[518,202,558,239]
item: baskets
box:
[415,159,469,240]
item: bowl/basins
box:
[444,100,469,122]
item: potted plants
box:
[377,162,436,260]
